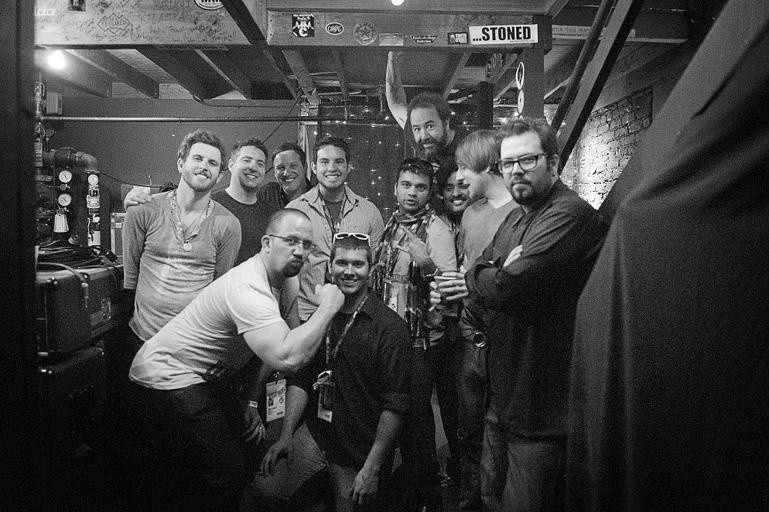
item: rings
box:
[259,427,263,430]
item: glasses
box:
[265,233,314,250]
[332,232,371,246]
[493,152,547,174]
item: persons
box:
[465,116,607,511]
[433,129,521,511]
[259,142,314,210]
[367,156,458,512]
[123,138,280,268]
[122,129,243,347]
[433,161,474,492]
[245,233,410,512]
[384,49,472,168]
[282,136,384,320]
[128,208,346,512]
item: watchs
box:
[245,401,258,407]
